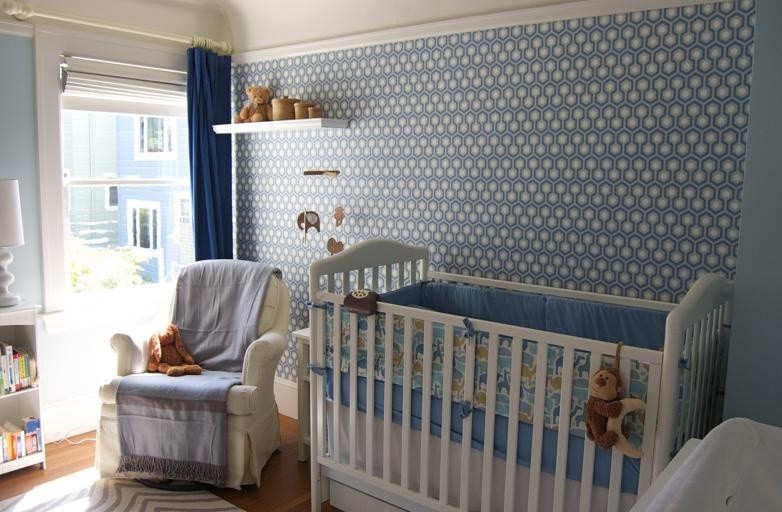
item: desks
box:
[293,326,310,464]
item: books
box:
[0,415,41,465]
[0,343,36,396]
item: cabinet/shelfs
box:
[0,306,46,476]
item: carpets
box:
[0,464,241,512]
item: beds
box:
[626,417,782,512]
[307,238,735,512]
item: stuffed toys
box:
[585,342,628,447]
[234,86,273,124]
[148,325,202,376]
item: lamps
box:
[0,178,26,308]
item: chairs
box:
[95,258,291,489]
